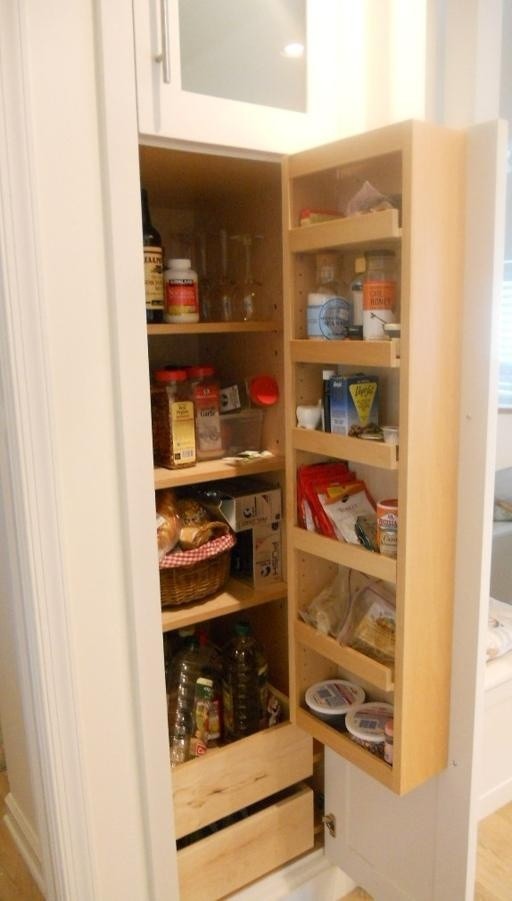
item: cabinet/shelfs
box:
[2,117,466,901]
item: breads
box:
[156,487,215,557]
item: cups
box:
[381,426,399,461]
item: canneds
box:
[382,719,394,765]
[361,249,397,340]
[376,499,398,557]
[156,364,227,471]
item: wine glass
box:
[184,231,263,321]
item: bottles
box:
[166,620,269,767]
[141,187,163,323]
[163,259,200,322]
[306,249,400,340]
[384,720,393,766]
